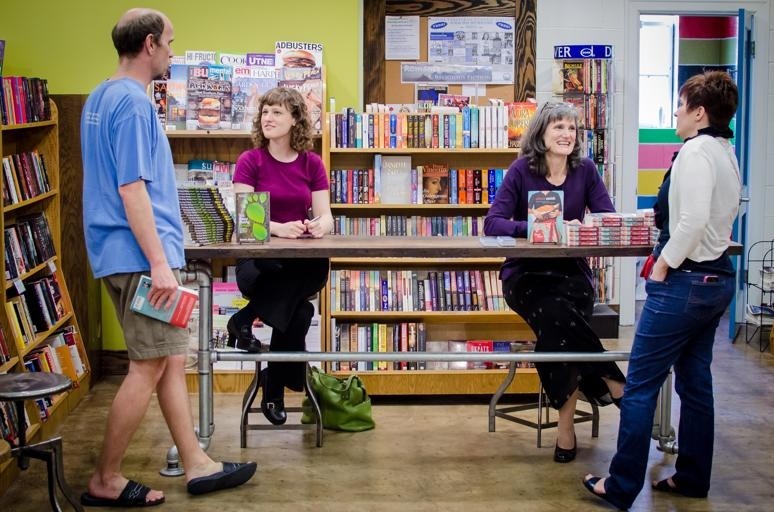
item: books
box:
[198,257,614,372]
[128,273,200,329]
[744,265,774,327]
[148,40,662,248]
[2,73,87,458]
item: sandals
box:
[583,471,629,510]
[653,477,679,492]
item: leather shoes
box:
[555,434,577,462]
[609,392,629,409]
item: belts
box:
[682,259,737,279]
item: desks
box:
[159,236,680,479]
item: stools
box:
[223,295,326,450]
[487,339,602,450]
[0,371,96,511]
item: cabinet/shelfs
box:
[741,238,774,355]
[322,109,606,406]
[146,62,327,400]
[0,93,94,495]
[45,92,106,390]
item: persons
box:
[482,98,628,464]
[76,5,260,509]
[482,32,489,40]
[580,69,743,512]
[492,32,501,42]
[507,33,513,40]
[225,85,336,426]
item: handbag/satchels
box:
[302,366,375,431]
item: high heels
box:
[226,312,261,353]
[259,368,287,424]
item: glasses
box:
[539,101,574,113]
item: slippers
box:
[187,462,258,494]
[81,481,163,507]
